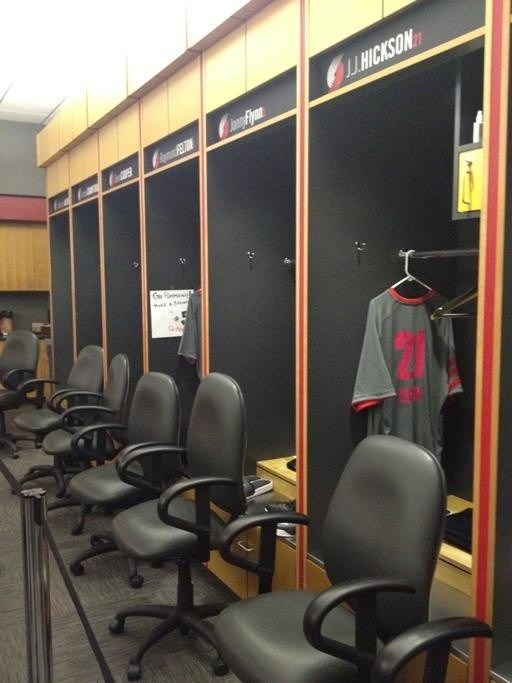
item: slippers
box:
[243,475,273,500]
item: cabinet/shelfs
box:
[60,102,108,435]
[86,81,144,468]
[187,0,304,600]
[36,113,75,411]
[485,0,512,682]
[126,28,205,503]
[299,0,503,682]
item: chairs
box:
[213,435,448,682]
[108,372,247,683]
[13,346,105,497]
[42,354,129,535]
[0,331,46,458]
[67,372,184,589]
[371,616,493,681]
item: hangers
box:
[390,251,433,290]
[430,286,477,321]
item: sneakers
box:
[276,522,296,538]
[264,500,296,512]
